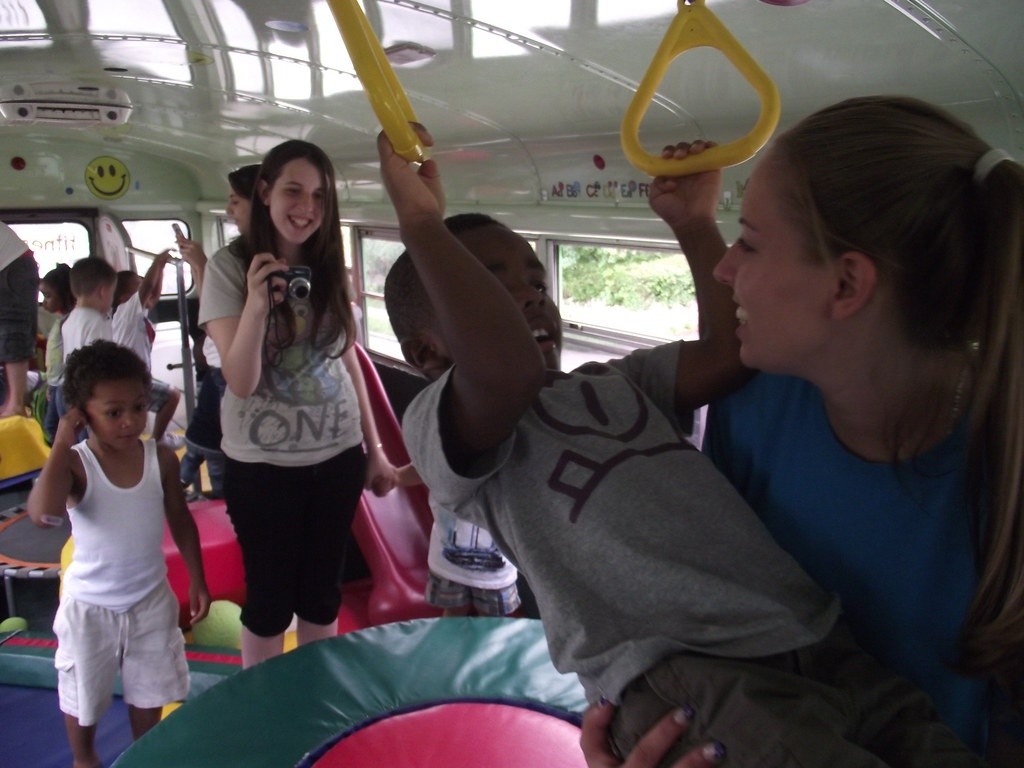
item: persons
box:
[375,122,991,768]
[1,220,40,418]
[113,246,178,444]
[196,142,399,670]
[26,338,210,768]
[178,371,227,501]
[40,263,87,444]
[372,459,523,616]
[56,257,120,446]
[174,164,264,396]
[580,97,1024,767]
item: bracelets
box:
[367,444,382,455]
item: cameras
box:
[261,265,312,302]
